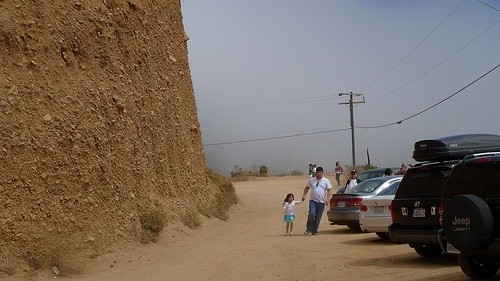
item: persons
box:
[308,164,316,178]
[382,163,412,176]
[283,193,306,235]
[344,170,362,194]
[302,167,332,236]
[335,161,343,186]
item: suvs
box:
[437,150,500,281]
[387,133,500,257]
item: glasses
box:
[316,182,319,186]
[352,174,357,175]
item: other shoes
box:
[304,230,319,236]
[285,232,292,235]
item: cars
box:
[326,173,403,231]
[336,165,400,193]
[359,176,405,239]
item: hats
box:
[351,170,357,174]
